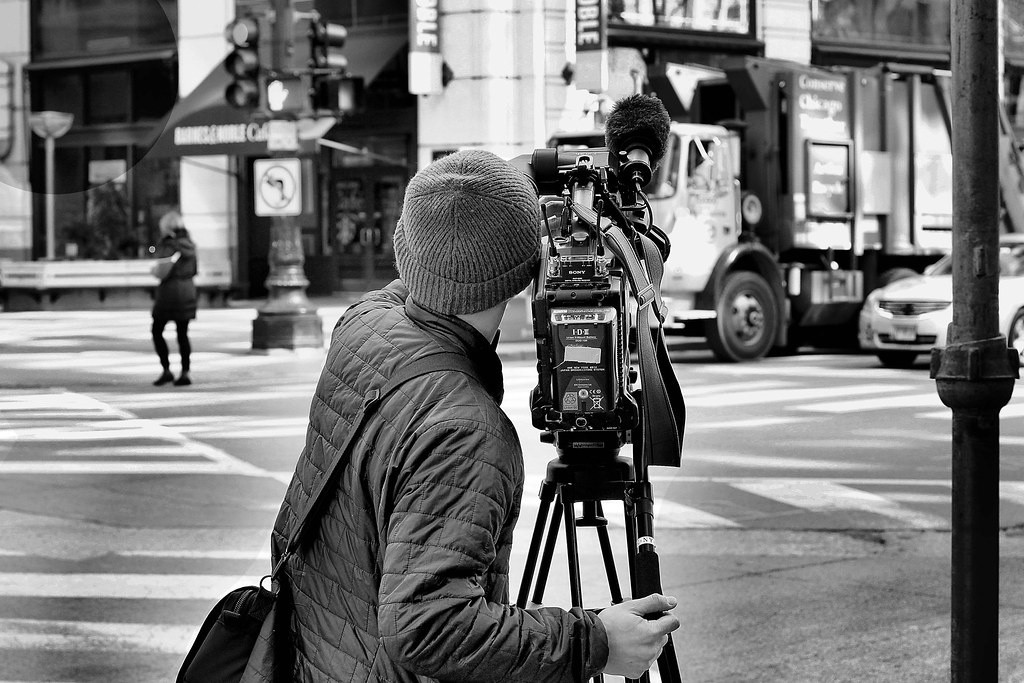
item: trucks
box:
[566,56,1024,365]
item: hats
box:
[393,149,542,316]
[159,211,185,238]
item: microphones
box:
[605,94,671,187]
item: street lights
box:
[25,110,81,257]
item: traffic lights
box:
[258,72,305,117]
[306,8,352,78]
[220,12,261,113]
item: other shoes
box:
[153,373,174,386]
[173,377,191,386]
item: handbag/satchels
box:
[174,585,278,683]
[150,250,182,280]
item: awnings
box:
[146,27,415,157]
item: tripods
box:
[515,423,663,683]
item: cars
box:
[858,231,1024,371]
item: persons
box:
[151,211,199,387]
[271,149,681,683]
